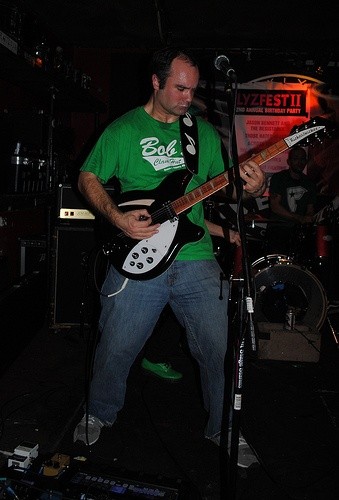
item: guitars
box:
[95,116,336,281]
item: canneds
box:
[283,311,295,330]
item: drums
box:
[235,255,329,331]
[300,222,339,270]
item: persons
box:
[268,149,315,258]
[203,198,260,272]
[73,49,266,468]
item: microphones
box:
[213,54,238,81]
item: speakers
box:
[57,184,107,226]
[51,225,98,330]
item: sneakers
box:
[141,358,183,379]
[211,428,259,470]
[73,412,104,446]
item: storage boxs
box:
[59,185,115,220]
[51,226,93,329]
[19,232,49,276]
[258,322,321,362]
